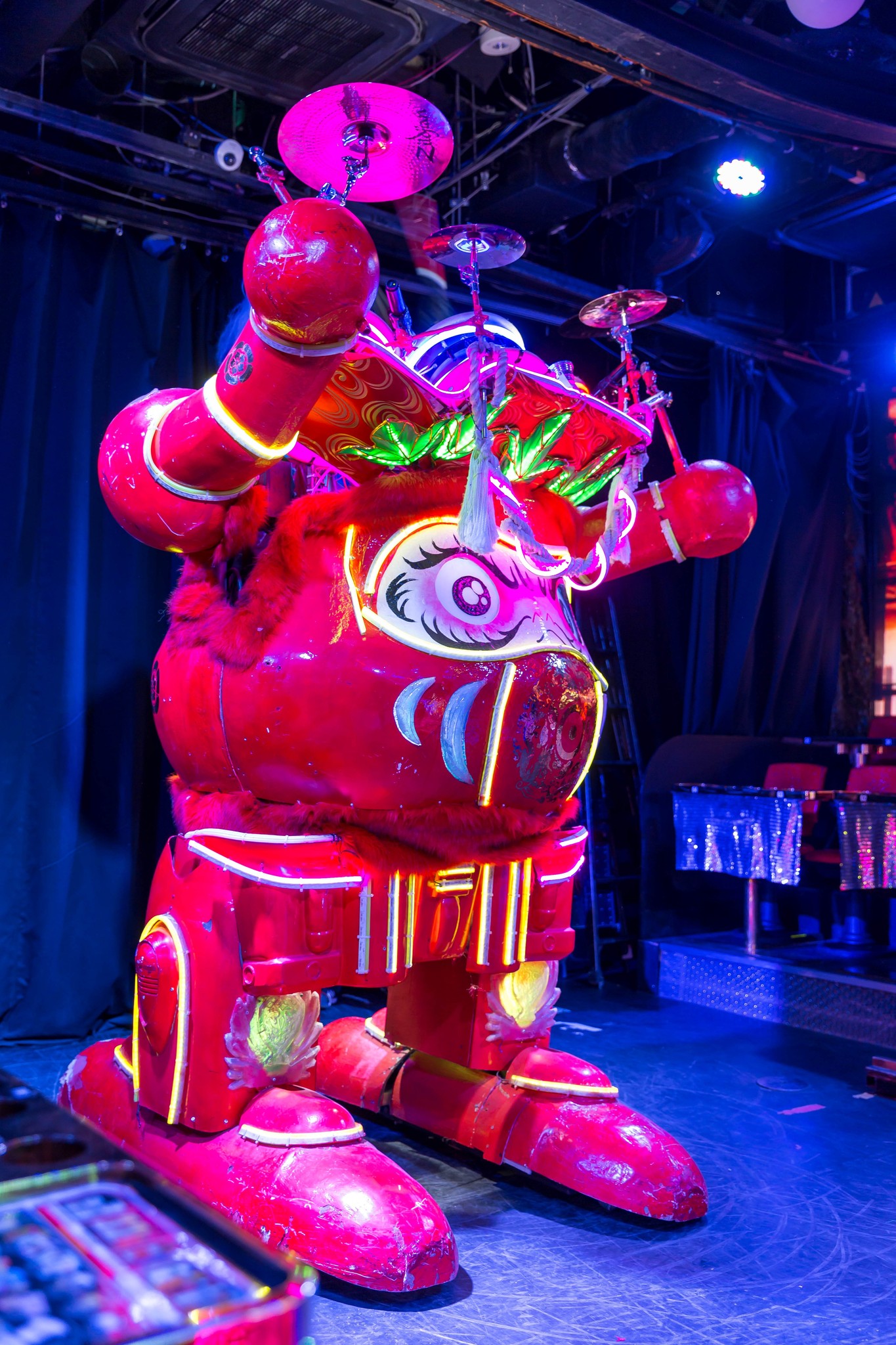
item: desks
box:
[669,782,896,955]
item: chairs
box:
[758,763,896,943]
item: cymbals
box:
[423,225,525,269]
[277,82,454,202]
[578,289,666,328]
[559,297,685,337]
